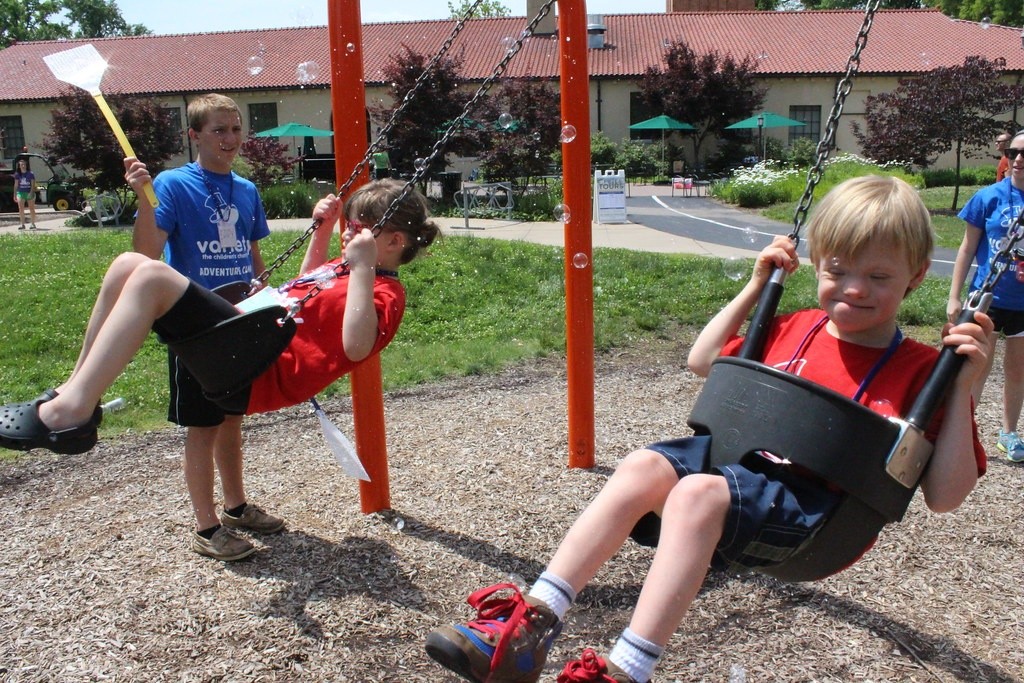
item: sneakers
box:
[193,526,255,561]
[996,429,1024,462]
[556,649,652,683]
[221,504,285,534]
[425,583,563,683]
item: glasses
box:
[995,141,1005,144]
[347,219,391,235]
[1004,149,1024,160]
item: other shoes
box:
[18,225,25,229]
[29,224,36,229]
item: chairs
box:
[671,164,724,197]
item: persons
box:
[425,175,994,683]
[13,160,36,229]
[0,178,437,456]
[372,145,392,181]
[124,93,286,561]
[946,130,1024,462]
[268,169,279,182]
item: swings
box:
[172,1,560,401]
[686,0,1024,578]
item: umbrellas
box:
[724,110,807,160]
[628,113,699,175]
[256,121,334,181]
[431,116,527,156]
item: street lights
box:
[758,114,764,160]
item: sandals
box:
[0,388,103,454]
